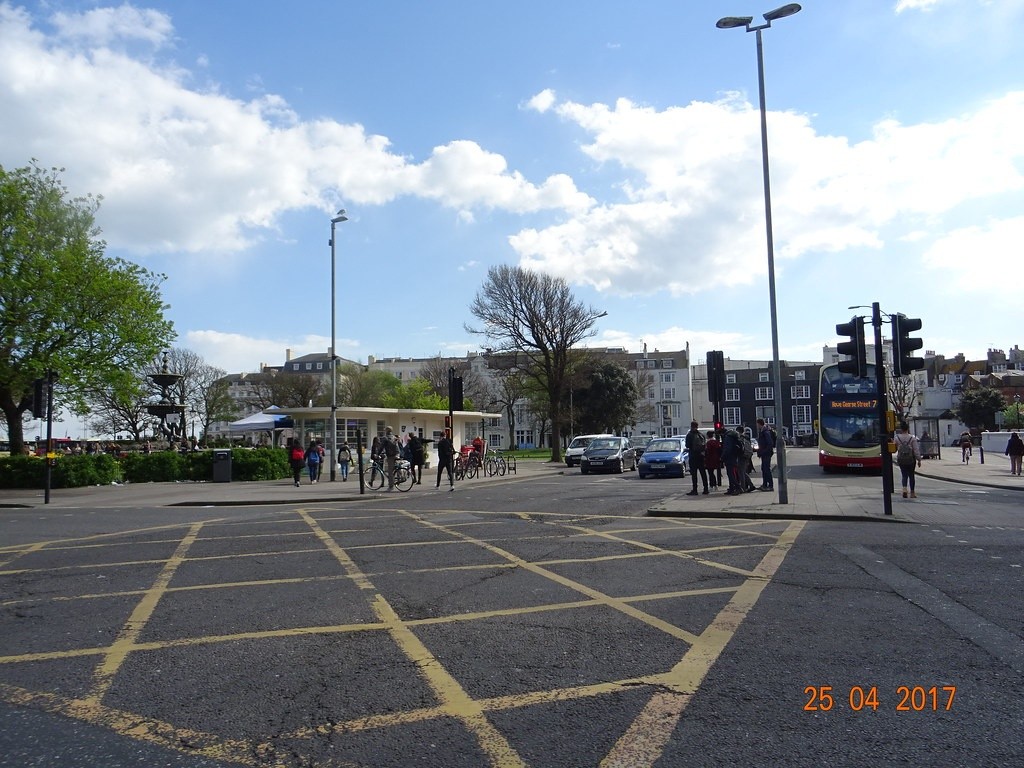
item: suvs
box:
[630,435,657,465]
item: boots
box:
[911,492,917,498]
[903,487,908,498]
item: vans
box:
[565,434,615,467]
[687,428,715,443]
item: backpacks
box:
[292,448,304,460]
[690,434,705,465]
[896,435,915,466]
[340,450,350,462]
[768,429,777,448]
[308,448,319,466]
[740,438,753,459]
[383,435,399,456]
[727,431,744,457]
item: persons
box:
[685,421,710,496]
[59,441,122,458]
[919,431,935,459]
[288,439,305,487]
[1005,433,1024,475]
[168,437,199,454]
[435,432,455,491]
[338,441,354,482]
[707,426,756,495]
[890,422,922,498]
[756,419,774,491]
[143,440,153,454]
[368,426,438,491]
[959,431,973,462]
[304,440,325,483]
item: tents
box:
[228,405,295,448]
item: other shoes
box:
[449,485,455,491]
[312,480,317,484]
[687,481,775,495]
[386,488,393,492]
[416,482,422,485]
[436,487,440,490]
[369,482,373,487]
[295,481,300,487]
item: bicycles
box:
[959,443,970,465]
[452,451,477,480]
[483,449,507,476]
[362,455,414,492]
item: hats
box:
[409,432,414,437]
[440,432,446,437]
[384,426,393,432]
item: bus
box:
[814,363,891,474]
[0,438,101,461]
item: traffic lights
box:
[891,314,924,377]
[835,314,867,377]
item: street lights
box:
[329,216,348,481]
[570,313,607,441]
[1013,393,1020,431]
[716,3,803,504]
[189,407,196,465]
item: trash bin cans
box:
[212,449,232,483]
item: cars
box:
[673,435,686,438]
[750,439,759,451]
[638,437,692,479]
[580,437,637,474]
